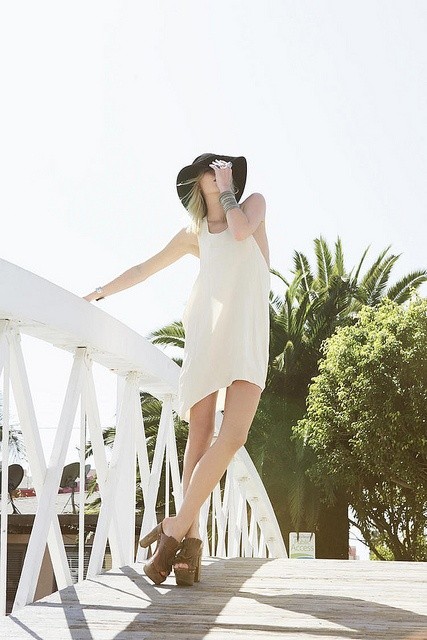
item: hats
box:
[176,153,247,212]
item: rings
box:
[213,161,233,171]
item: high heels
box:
[140,522,181,584]
[173,537,203,586]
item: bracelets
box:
[96,287,104,302]
[218,191,239,214]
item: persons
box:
[82,152,275,587]
